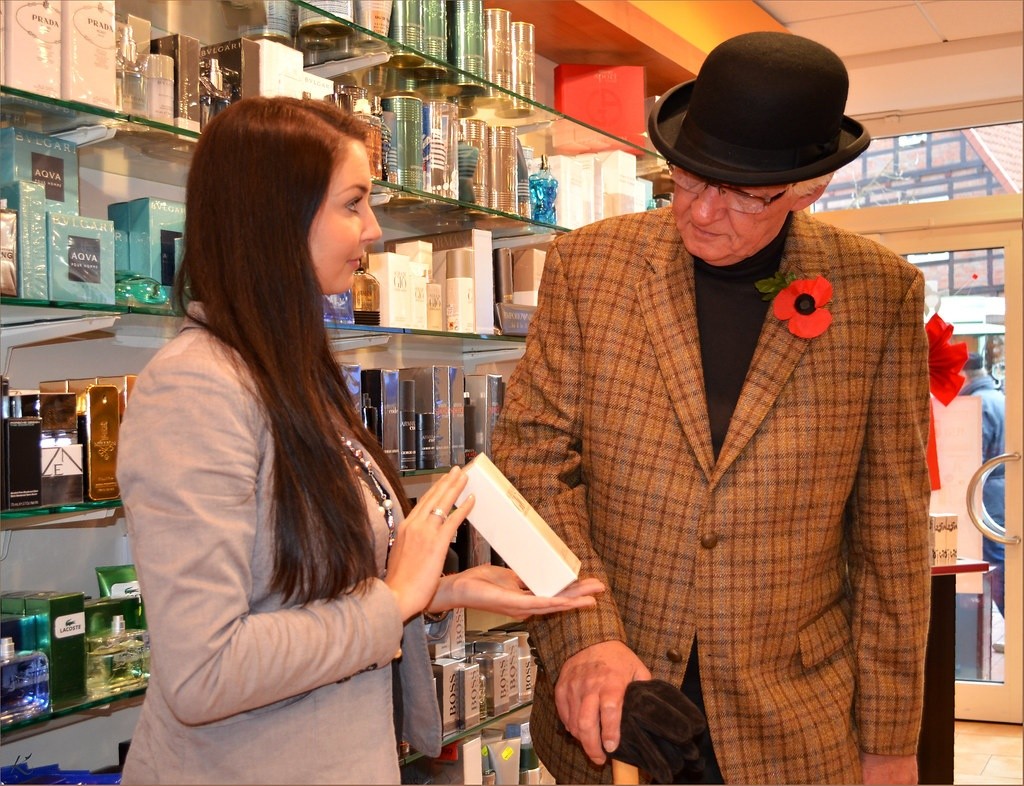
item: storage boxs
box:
[931,512,959,568]
[555,61,650,159]
[2,3,583,783]
[0,0,656,785]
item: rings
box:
[428,509,447,521]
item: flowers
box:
[755,272,832,338]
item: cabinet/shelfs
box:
[0,0,701,786]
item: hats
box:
[648,32,871,186]
[962,352,983,370]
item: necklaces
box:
[337,430,395,548]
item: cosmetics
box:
[0,0,676,786]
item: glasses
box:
[665,161,800,214]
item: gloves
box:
[556,678,711,786]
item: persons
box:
[490,32,931,786]
[113,96,605,786]
[956,353,1006,655]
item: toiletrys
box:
[487,737,523,786]
